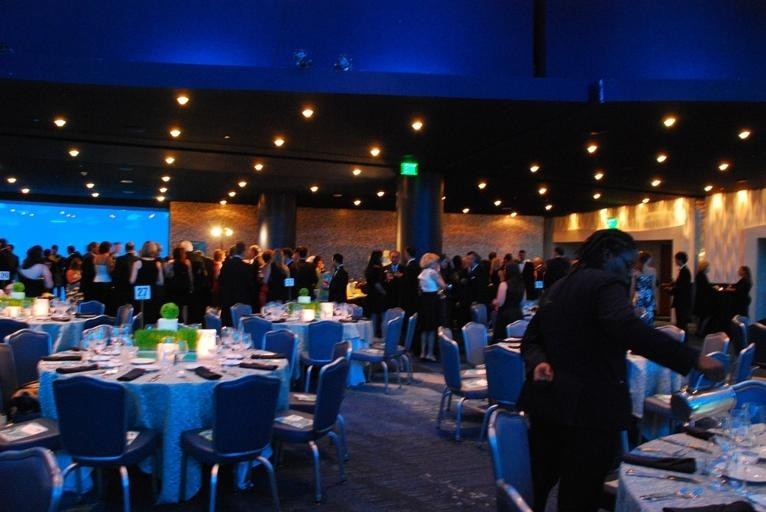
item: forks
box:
[636,486,702,504]
[636,445,689,459]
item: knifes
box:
[656,434,710,454]
[621,467,702,488]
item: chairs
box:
[439,321,489,444]
[473,299,764,510]
[2,299,379,510]
[347,307,417,396]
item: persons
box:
[0,237,350,328]
[362,244,754,363]
[514,229,726,512]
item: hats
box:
[419,253,441,268]
[178,240,194,252]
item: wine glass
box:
[261,298,353,321]
[51,298,79,319]
[703,399,766,502]
[78,323,253,378]
